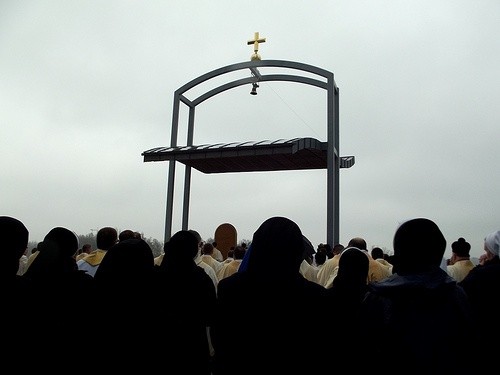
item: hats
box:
[450,236,472,259]
[485,230,500,256]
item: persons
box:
[0,205,500,375]
